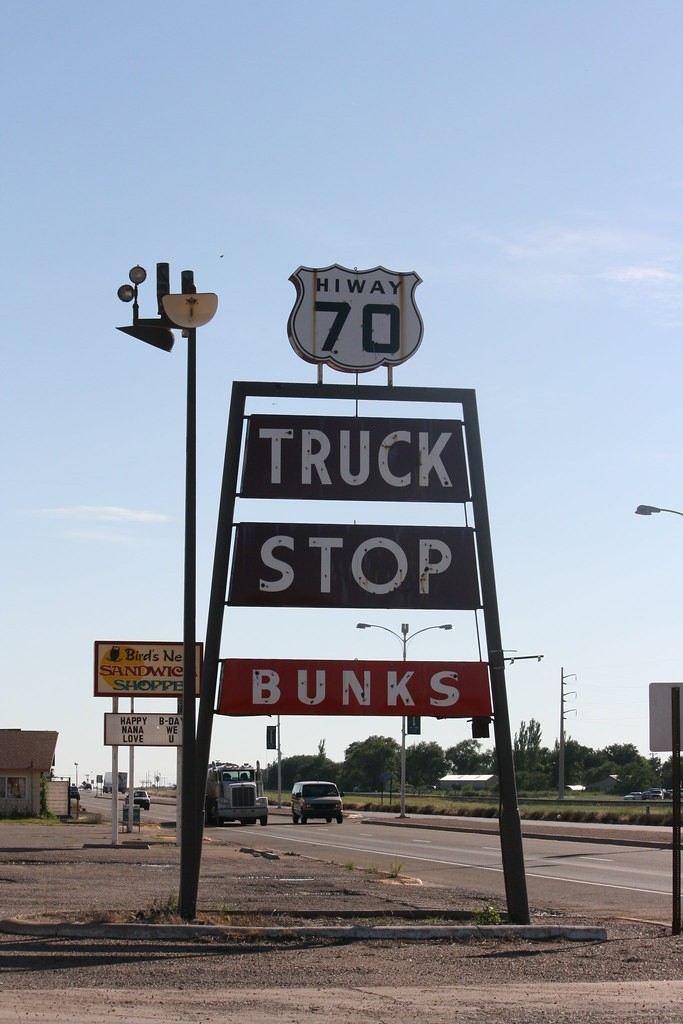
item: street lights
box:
[354,622,453,821]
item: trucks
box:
[203,759,268,827]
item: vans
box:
[291,780,345,824]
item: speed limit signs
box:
[287,263,424,374]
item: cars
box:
[126,790,151,810]
[69,786,79,799]
